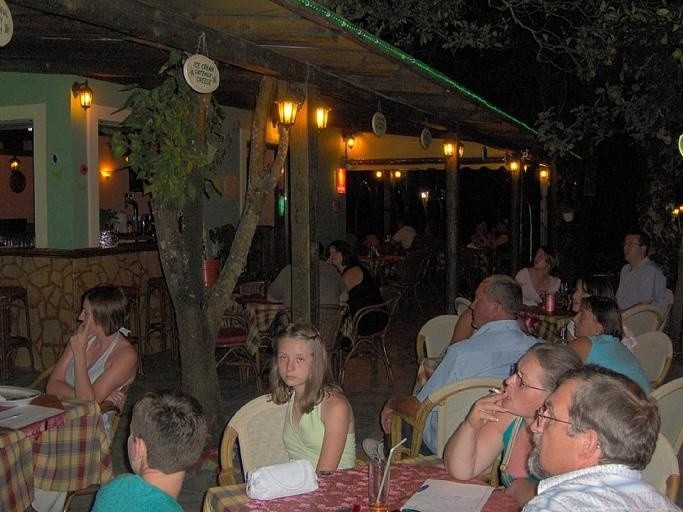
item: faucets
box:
[124,197,138,231]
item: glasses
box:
[510,364,546,393]
[536,406,576,431]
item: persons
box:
[559,274,639,353]
[362,274,543,464]
[260,203,513,352]
[614,228,667,316]
[518,364,683,512]
[259,317,357,474]
[566,295,655,399]
[514,244,567,307]
[87,388,208,512]
[444,340,583,510]
[24,285,138,512]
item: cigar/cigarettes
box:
[489,386,504,396]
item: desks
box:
[518,304,578,343]
[205,454,522,510]
[1,386,98,512]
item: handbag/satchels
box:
[245,458,320,501]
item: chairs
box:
[391,377,504,461]
[212,279,292,394]
[120,276,179,375]
[317,285,404,391]
[31,363,131,441]
[1,285,36,385]
[621,289,682,506]
[415,297,472,394]
[219,392,290,485]
[355,240,620,306]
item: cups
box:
[545,293,556,313]
[367,459,390,512]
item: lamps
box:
[8,154,21,176]
[72,77,94,112]
[312,94,332,135]
[458,142,464,160]
[336,167,346,194]
[442,132,454,160]
[274,85,308,128]
[342,128,354,150]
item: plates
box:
[0,387,43,407]
